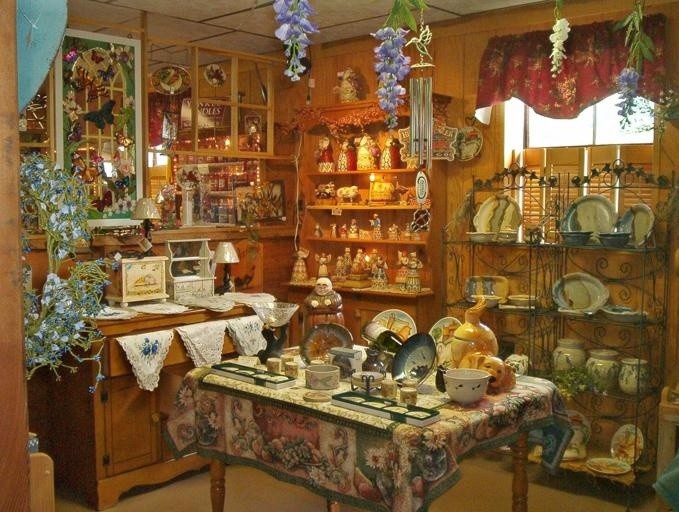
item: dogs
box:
[458,351,507,388]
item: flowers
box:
[20,154,122,396]
[369,0,428,129]
[611,1,679,138]
[548,0,572,76]
[272,0,320,84]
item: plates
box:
[299,322,354,366]
[585,457,633,475]
[551,272,611,315]
[560,193,617,232]
[464,275,509,305]
[620,203,657,248]
[610,424,644,465]
[565,409,592,448]
[472,194,522,232]
[427,316,462,350]
[390,331,436,389]
[367,309,417,346]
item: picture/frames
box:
[52,25,145,230]
[232,178,286,226]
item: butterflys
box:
[60,43,137,211]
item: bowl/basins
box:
[496,230,518,245]
[507,294,540,306]
[559,229,632,249]
[466,231,494,244]
[470,294,502,309]
[443,368,492,407]
[252,302,300,327]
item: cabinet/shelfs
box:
[560,159,676,507]
[446,160,562,484]
[278,91,453,356]
[47,292,280,511]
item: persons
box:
[314,247,424,293]
[304,278,344,328]
[350,218,358,234]
[368,213,381,232]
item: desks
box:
[186,343,556,512]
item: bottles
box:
[361,320,405,353]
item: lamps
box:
[212,241,239,293]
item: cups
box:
[281,354,294,371]
[400,387,418,406]
[380,372,398,400]
[285,362,299,379]
[267,357,282,374]
